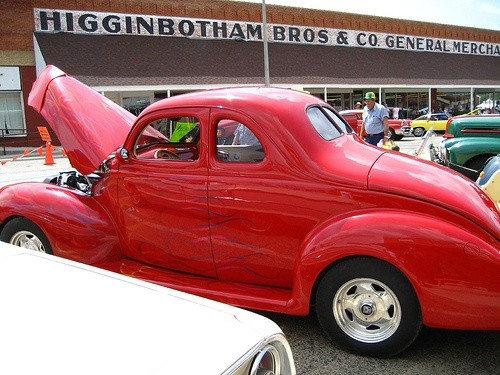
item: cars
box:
[0,239,298,375]
[409,113,452,137]
[427,114,500,213]
[0,86,500,358]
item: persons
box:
[412,108,418,118]
[360,92,390,146]
[382,102,399,119]
[432,100,469,119]
[496,101,500,112]
[232,123,258,146]
[355,102,362,120]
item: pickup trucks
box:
[337,107,414,142]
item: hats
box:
[354,102,363,106]
[364,92,376,99]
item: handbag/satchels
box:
[382,140,400,152]
[377,139,383,148]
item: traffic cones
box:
[43,141,56,165]
[61,147,68,158]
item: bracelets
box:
[384,136,390,140]
